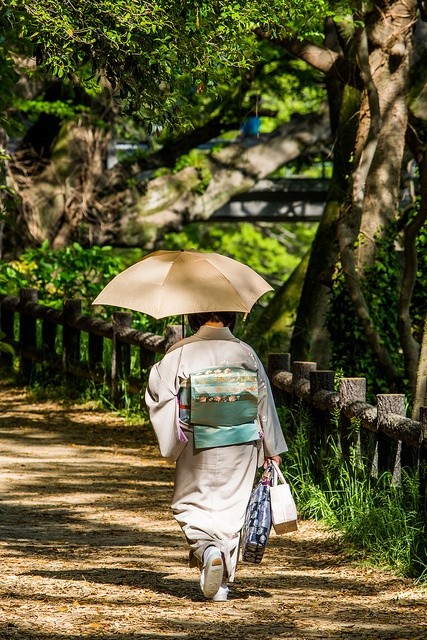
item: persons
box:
[144,311,288,602]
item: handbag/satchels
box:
[242,459,272,563]
[270,460,298,535]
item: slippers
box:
[199,545,224,599]
[213,583,230,602]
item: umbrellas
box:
[91,250,275,321]
